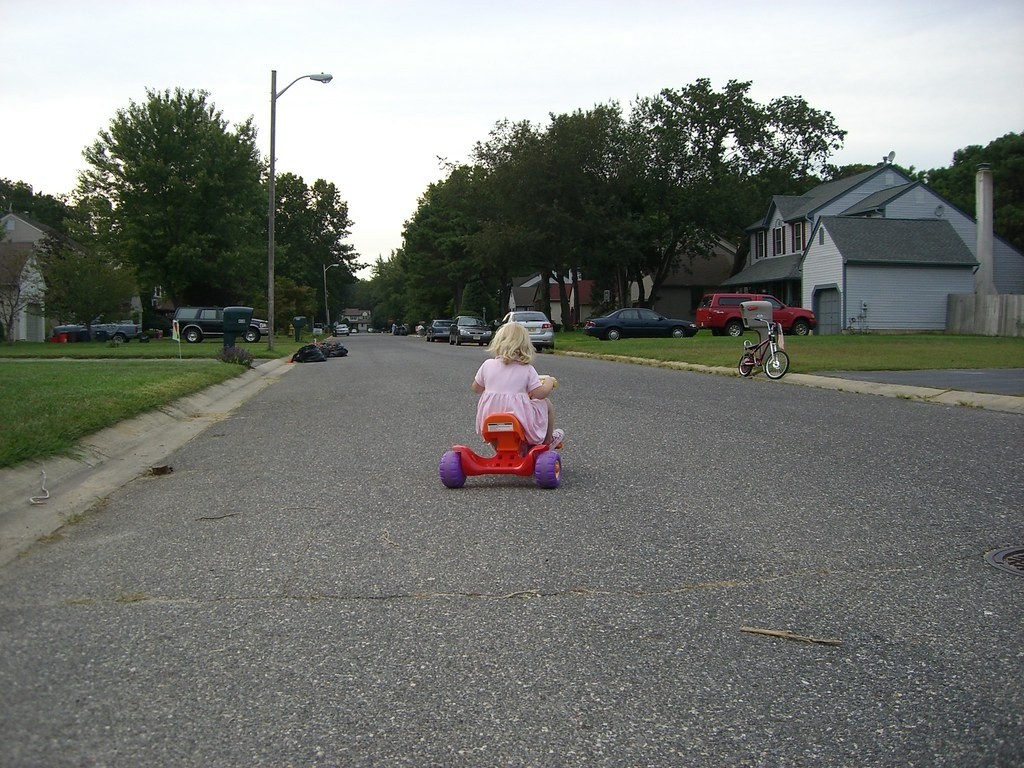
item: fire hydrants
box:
[288,324,294,335]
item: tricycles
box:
[440,375,563,489]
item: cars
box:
[449,316,492,346]
[426,320,453,342]
[583,308,698,341]
[53,317,142,343]
[494,311,554,351]
[336,324,349,336]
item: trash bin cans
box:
[58,331,90,343]
[95,330,107,342]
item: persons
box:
[471,323,565,451]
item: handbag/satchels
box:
[777,323,784,350]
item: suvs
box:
[173,307,269,343]
[697,293,816,337]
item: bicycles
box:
[738,318,790,379]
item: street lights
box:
[323,264,339,324]
[267,70,333,351]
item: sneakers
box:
[548,429,565,450]
